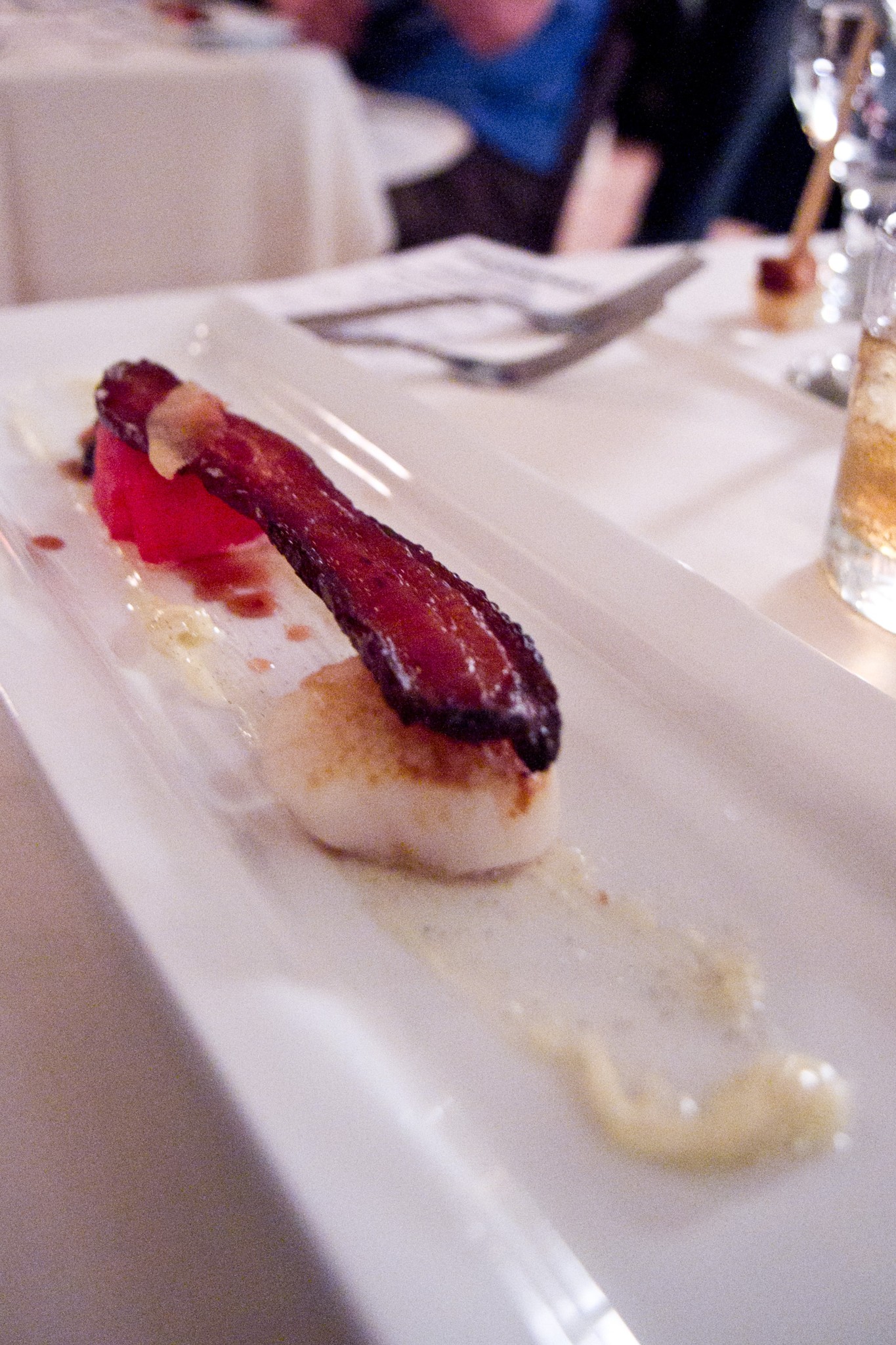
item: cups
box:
[819,210,895,637]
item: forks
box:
[314,294,667,392]
[290,255,704,338]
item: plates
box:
[0,236,896,1345]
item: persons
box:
[135,0,834,261]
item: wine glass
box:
[783,0,896,409]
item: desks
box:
[0,225,896,1345]
[0,0,397,297]
